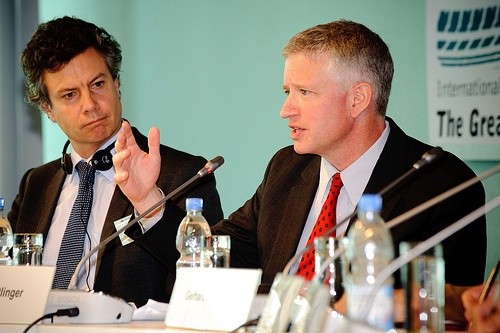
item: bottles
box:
[0,198,15,266]
[175,198,213,269]
[350,195,395,333]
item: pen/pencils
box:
[466,261,500,331]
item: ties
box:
[295,173,343,296]
[51,160,95,290]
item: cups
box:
[311,238,350,303]
[400,240,444,333]
[201,235,230,269]
[13,233,44,266]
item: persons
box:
[112,19,487,333]
[0,14,223,301]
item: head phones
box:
[61,117,131,174]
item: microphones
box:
[255,145,443,333]
[45,155,224,321]
